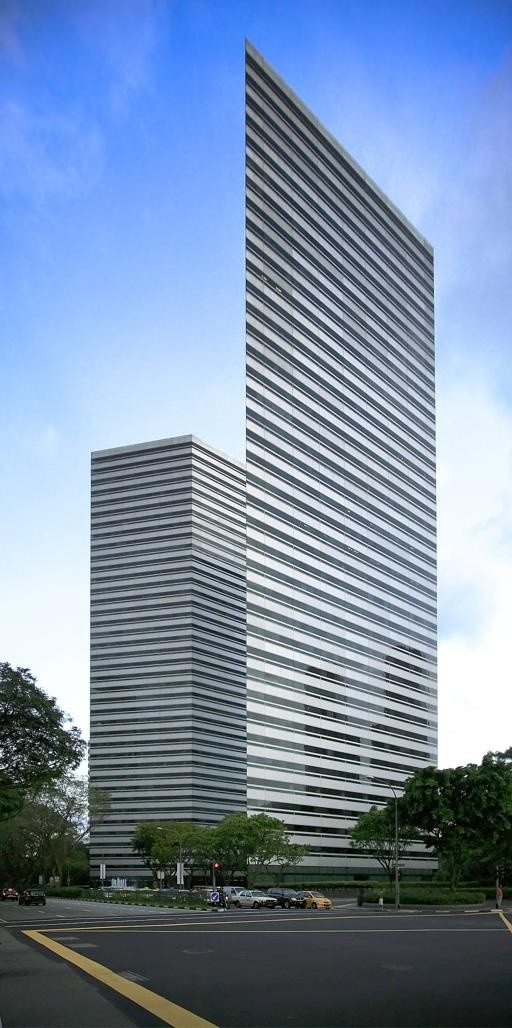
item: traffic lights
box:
[214,862,219,876]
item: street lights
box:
[156,825,182,889]
[365,773,401,909]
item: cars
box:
[17,887,46,905]
[191,883,333,911]
[0,887,19,901]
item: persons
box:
[377,888,385,910]
[495,882,505,909]
